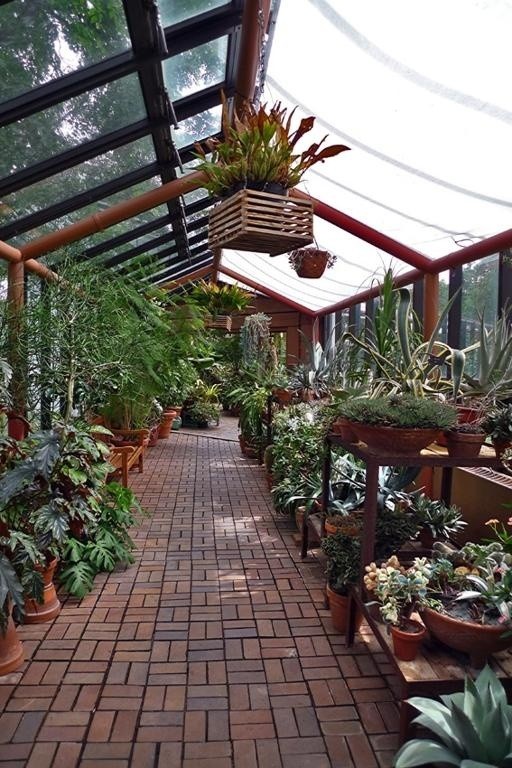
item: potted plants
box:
[415,518,512,670]
[85,259,222,448]
[184,86,351,230]
[374,558,435,661]
[287,247,338,279]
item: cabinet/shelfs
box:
[345,589,512,754]
